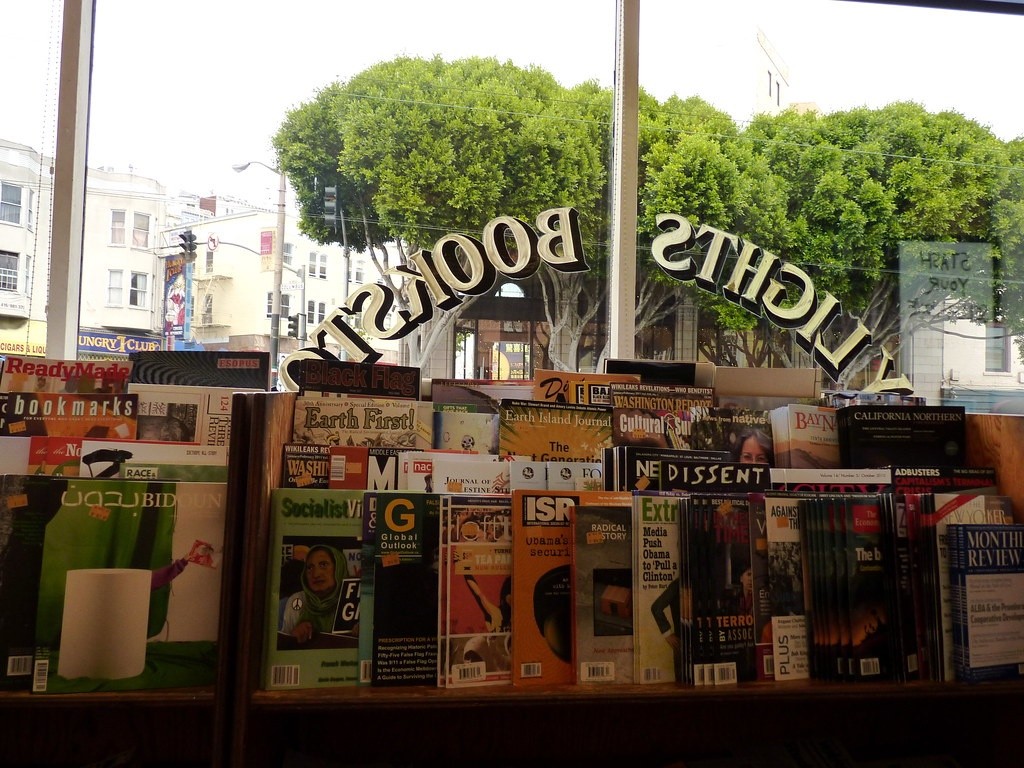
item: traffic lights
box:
[180,231,198,261]
[287,315,298,338]
[323,185,337,230]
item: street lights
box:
[232,161,287,390]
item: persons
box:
[651,577,680,681]
[733,429,775,464]
[726,564,753,614]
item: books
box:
[0,351,1024,690]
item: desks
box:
[0,687,1024,768]
